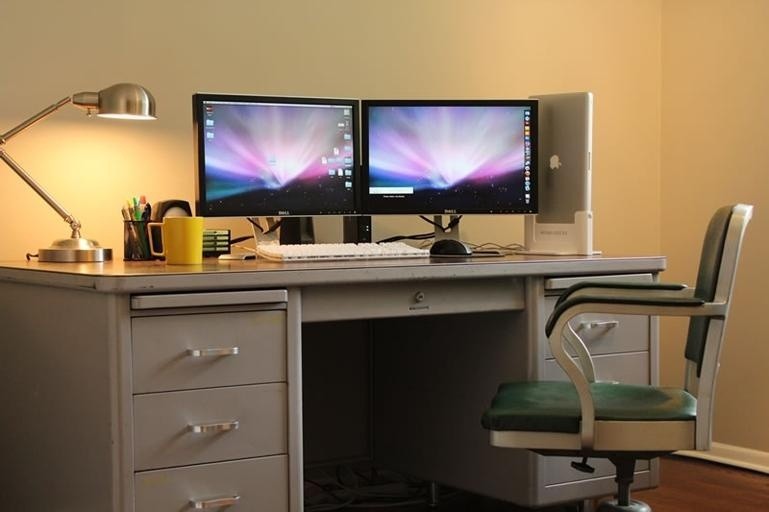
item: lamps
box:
[0,82,158,263]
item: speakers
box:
[151,200,191,260]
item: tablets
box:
[525,90,595,255]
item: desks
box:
[0,255,666,511]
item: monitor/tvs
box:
[191,93,360,242]
[362,99,540,255]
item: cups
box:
[146,215,205,266]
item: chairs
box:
[482,204,755,512]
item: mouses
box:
[430,238,474,259]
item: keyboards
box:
[253,241,429,264]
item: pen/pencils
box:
[121,196,153,260]
